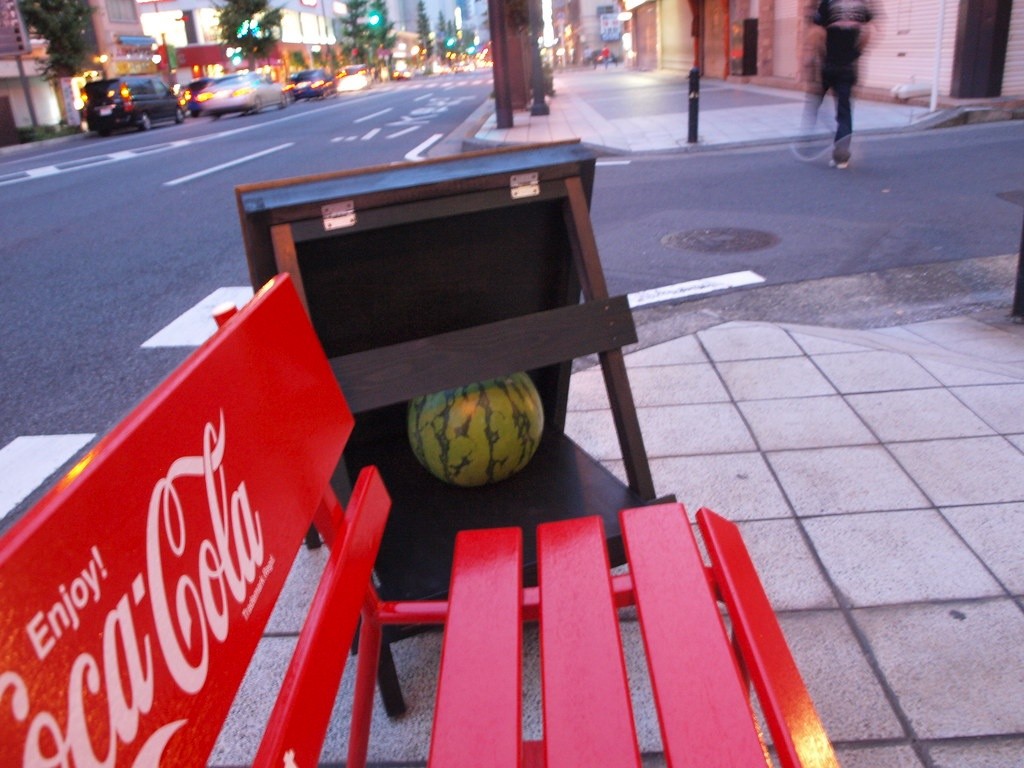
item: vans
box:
[86,75,183,138]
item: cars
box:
[291,70,337,102]
[193,72,288,119]
[187,77,213,109]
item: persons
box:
[583,44,610,72]
[793,1,875,169]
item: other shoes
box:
[829,158,848,170]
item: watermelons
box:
[408,371,544,486]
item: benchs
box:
[0,268,847,767]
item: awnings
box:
[118,35,157,46]
